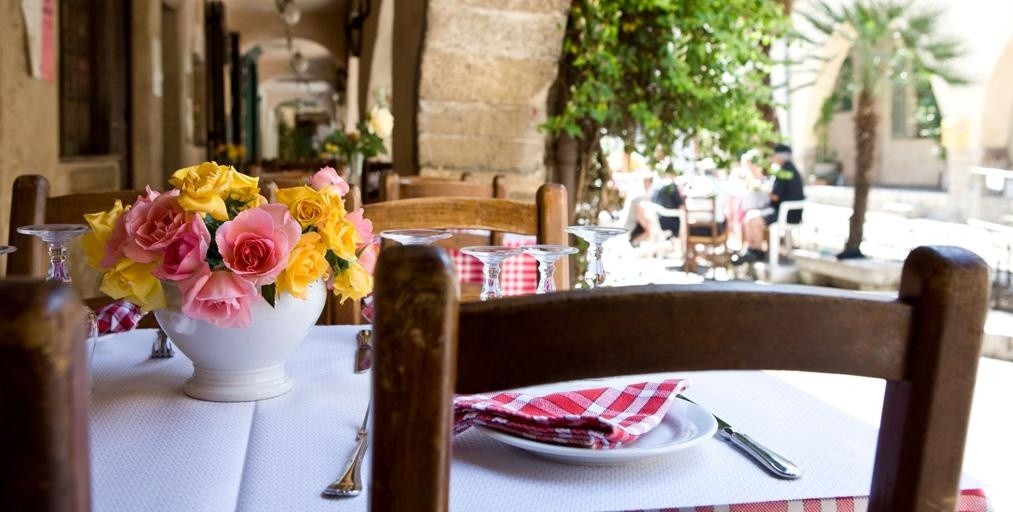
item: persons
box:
[730,141,805,265]
[629,175,727,248]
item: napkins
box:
[453,378,689,451]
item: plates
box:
[470,395,720,468]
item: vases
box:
[151,281,327,404]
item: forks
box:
[150,326,176,358]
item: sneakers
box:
[732,250,764,265]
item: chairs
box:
[372,240,992,510]
[245,157,822,283]
[335,182,573,328]
[2,174,184,345]
[1,280,92,512]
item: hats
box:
[775,145,790,152]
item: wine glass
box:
[14,222,100,400]
[372,216,629,297]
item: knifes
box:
[675,394,803,481]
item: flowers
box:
[63,159,380,312]
